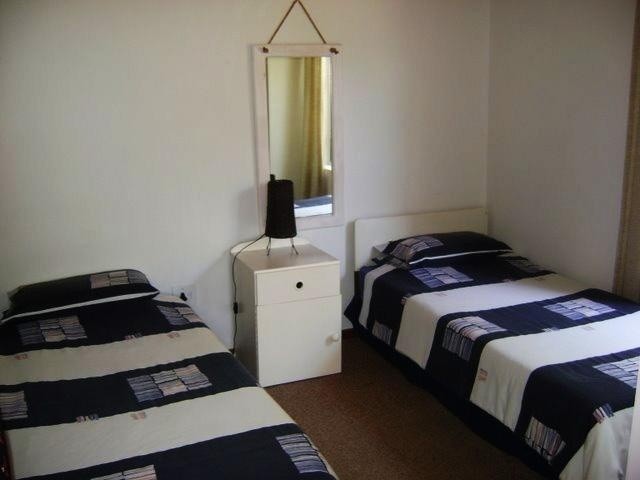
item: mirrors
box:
[253,44,345,230]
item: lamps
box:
[266,179,299,255]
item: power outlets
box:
[173,285,196,305]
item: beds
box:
[0,295,339,480]
[294,194,333,218]
[345,206,640,480]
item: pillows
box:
[375,230,513,264]
[3,269,159,321]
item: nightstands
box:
[230,235,342,387]
